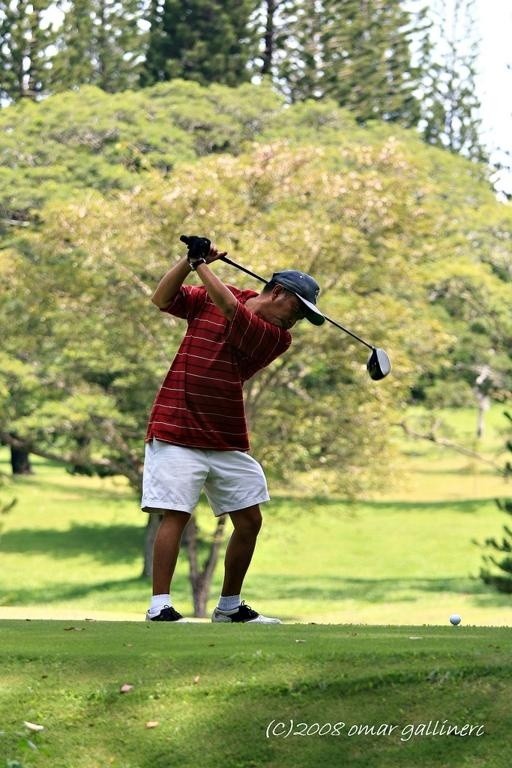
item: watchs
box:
[189,258,206,270]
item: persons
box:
[138,234,327,625]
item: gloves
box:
[187,235,211,270]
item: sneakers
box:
[211,599,281,624]
[145,603,192,622]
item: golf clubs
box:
[179,234,390,381]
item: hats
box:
[271,269,325,325]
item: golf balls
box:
[450,615,461,626]
